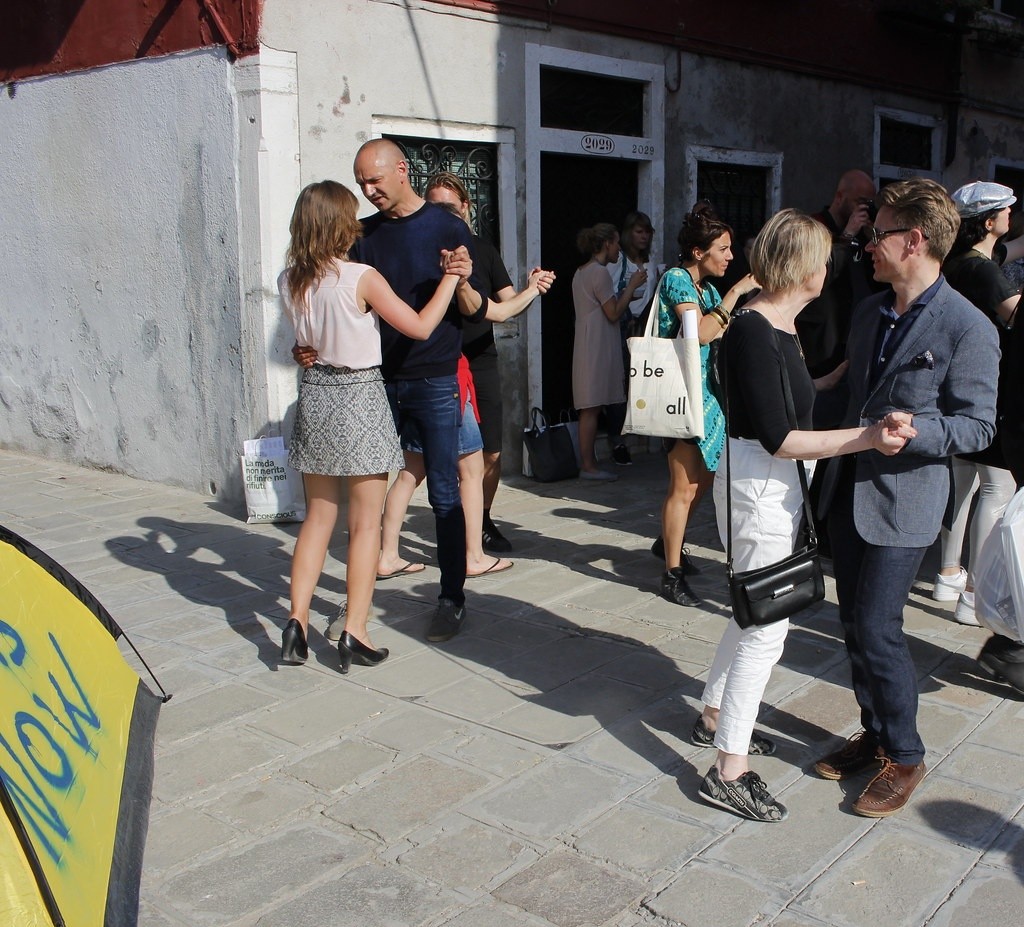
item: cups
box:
[658,264,667,275]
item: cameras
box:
[861,203,879,222]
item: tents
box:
[0,526,174,927]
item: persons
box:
[378,170,555,579]
[690,208,917,822]
[606,210,668,464]
[813,175,1003,816]
[743,169,1024,696]
[570,223,648,481]
[277,179,461,671]
[651,209,762,606]
[291,138,488,642]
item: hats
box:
[949,180,1017,218]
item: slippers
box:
[377,561,426,578]
[465,557,514,578]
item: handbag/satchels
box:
[620,270,706,441]
[522,408,577,481]
[241,428,309,525]
[726,544,826,630]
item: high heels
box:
[281,618,308,664]
[338,627,389,673]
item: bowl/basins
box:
[633,290,646,297]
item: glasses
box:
[871,227,930,245]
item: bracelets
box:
[710,305,730,327]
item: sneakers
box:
[612,446,633,466]
[427,596,467,642]
[851,748,927,818]
[690,717,776,756]
[956,593,983,627]
[329,598,374,640]
[815,730,892,778]
[660,566,702,609]
[933,566,971,602]
[482,515,513,553]
[699,764,789,823]
[652,534,700,574]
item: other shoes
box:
[646,457,670,480]
[977,636,1024,694]
[580,467,615,480]
